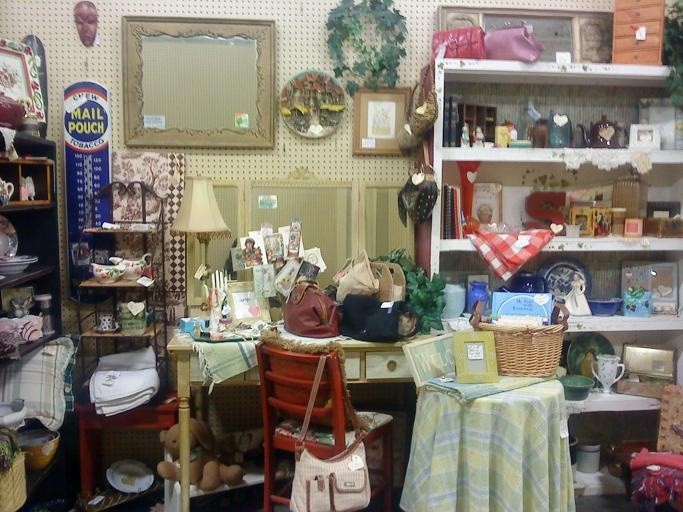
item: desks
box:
[167,321,436,512]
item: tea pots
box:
[577,114,629,148]
[109,253,152,280]
[500,271,547,293]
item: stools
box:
[75,393,179,503]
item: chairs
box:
[255,341,396,512]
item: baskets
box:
[471,292,570,378]
[0,428,27,512]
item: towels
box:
[89,347,159,417]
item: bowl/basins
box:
[22,435,61,470]
[587,300,622,317]
[558,375,595,401]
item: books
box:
[444,95,458,145]
[442,182,463,239]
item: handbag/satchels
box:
[486,20,544,65]
[431,16,485,63]
[289,355,370,512]
[397,141,440,228]
[399,84,426,148]
[341,261,405,341]
[409,65,439,135]
[284,278,340,338]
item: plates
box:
[19,429,60,445]
[0,216,18,258]
[0,255,38,263]
[106,460,154,493]
[0,260,38,275]
[93,322,119,332]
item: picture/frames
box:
[449,328,500,385]
[634,94,683,152]
[472,181,506,232]
[624,121,661,153]
[0,39,45,125]
[121,16,275,149]
[353,87,410,157]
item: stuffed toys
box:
[156,419,245,491]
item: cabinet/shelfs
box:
[78,181,170,389]
[415,60,683,497]
[0,133,64,512]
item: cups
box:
[495,125,511,148]
[0,178,13,206]
[100,314,113,330]
[591,355,624,395]
[566,225,579,238]
[91,263,126,283]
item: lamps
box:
[168,176,232,320]
